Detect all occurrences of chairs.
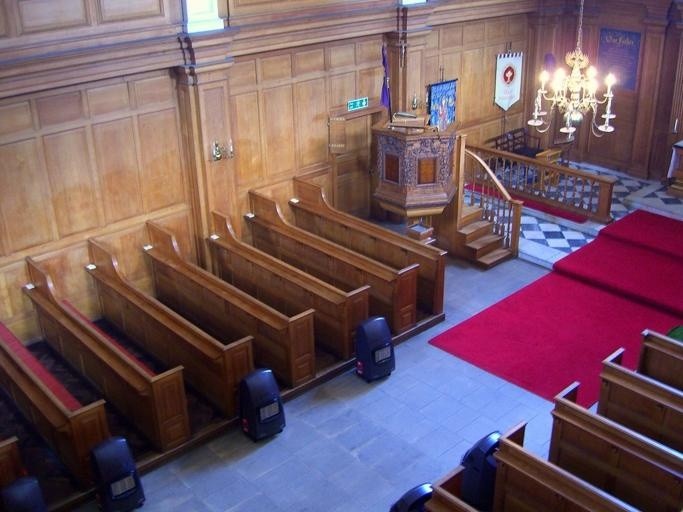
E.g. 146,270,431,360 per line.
513,129,541,175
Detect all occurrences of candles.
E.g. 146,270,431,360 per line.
673,117,677,131
533,64,613,129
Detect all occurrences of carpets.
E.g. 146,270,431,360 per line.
428,209,683,409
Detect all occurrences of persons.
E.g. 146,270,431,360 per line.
435,96,450,131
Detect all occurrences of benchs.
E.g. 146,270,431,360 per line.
388,328,683,512
0,177,447,512
